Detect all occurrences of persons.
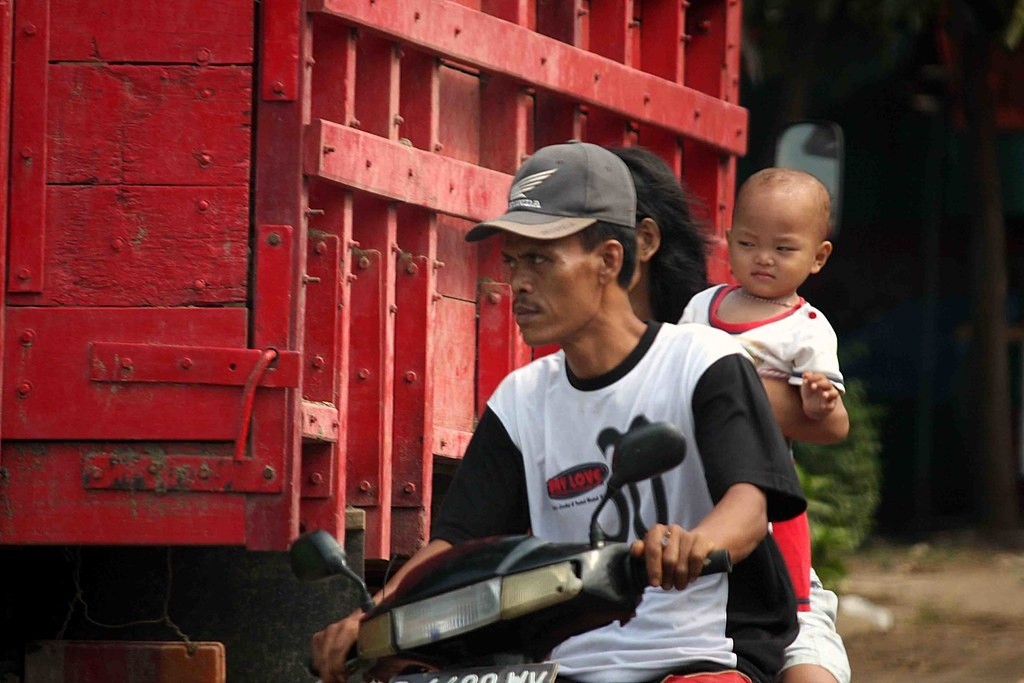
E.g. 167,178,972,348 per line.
309,140,852,683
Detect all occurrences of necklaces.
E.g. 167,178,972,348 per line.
736,286,797,308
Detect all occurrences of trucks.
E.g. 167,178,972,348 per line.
0,0,845,683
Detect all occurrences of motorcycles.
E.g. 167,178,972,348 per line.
285,526,730,683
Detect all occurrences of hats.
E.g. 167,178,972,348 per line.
462,139,638,238
